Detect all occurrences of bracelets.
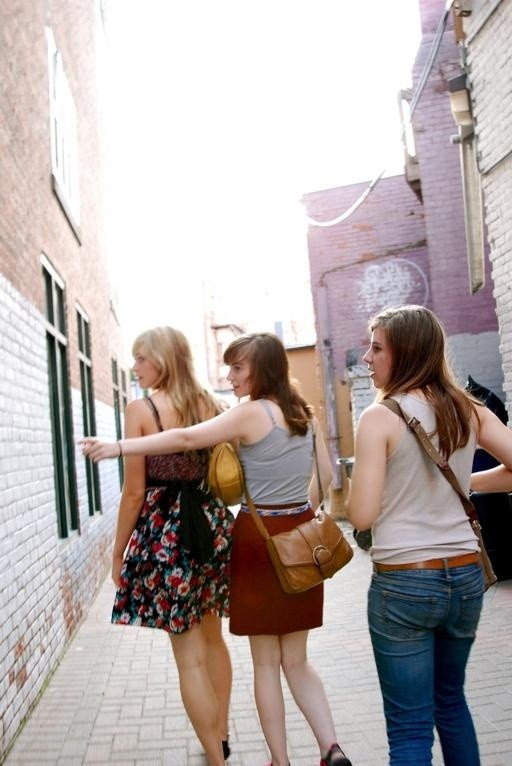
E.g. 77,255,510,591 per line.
117,440,123,458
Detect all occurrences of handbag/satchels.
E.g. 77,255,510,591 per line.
266,510,353,595
470,512,498,593
207,441,244,506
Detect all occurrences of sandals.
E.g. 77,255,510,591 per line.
321,743,352,766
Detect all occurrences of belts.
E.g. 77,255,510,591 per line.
373,552,479,570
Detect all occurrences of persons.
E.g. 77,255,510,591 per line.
110,325,234,766
76,332,353,766
346,301,512,765
